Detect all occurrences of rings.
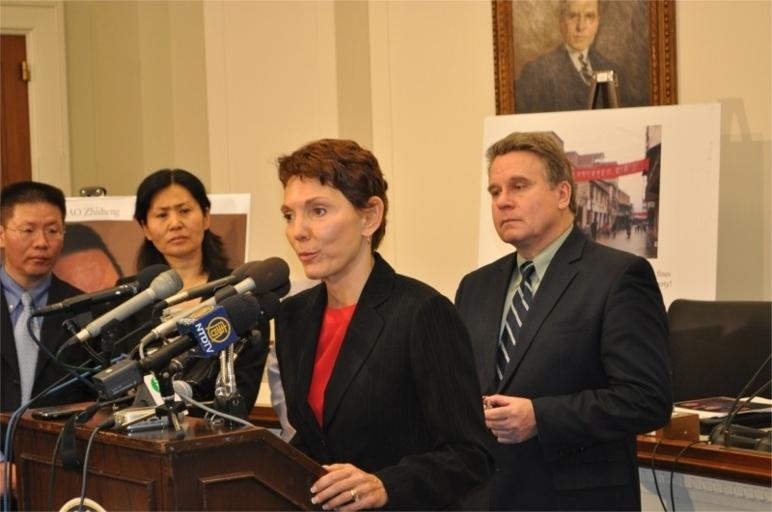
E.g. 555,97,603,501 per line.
351,490,359,502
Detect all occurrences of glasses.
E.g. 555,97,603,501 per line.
6,224,65,240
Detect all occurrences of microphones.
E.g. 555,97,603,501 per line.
30,262,170,318
155,259,261,310
150,256,290,340
75,269,185,344
272,281,291,300
708,352,772,452
167,291,282,374
92,294,264,403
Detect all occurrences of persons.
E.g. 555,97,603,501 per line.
51,222,125,293
515,0,631,115
455,132,674,512
105,168,270,426
0,181,106,512
274,138,493,511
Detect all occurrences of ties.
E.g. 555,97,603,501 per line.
13,292,40,408
494,261,535,389
579,54,593,86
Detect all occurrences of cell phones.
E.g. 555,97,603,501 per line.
31,405,85,421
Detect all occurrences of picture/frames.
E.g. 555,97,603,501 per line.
491,0,678,119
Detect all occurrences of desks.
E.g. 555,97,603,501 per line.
637,402,771,512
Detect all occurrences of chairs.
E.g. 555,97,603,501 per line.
666,297,772,403
